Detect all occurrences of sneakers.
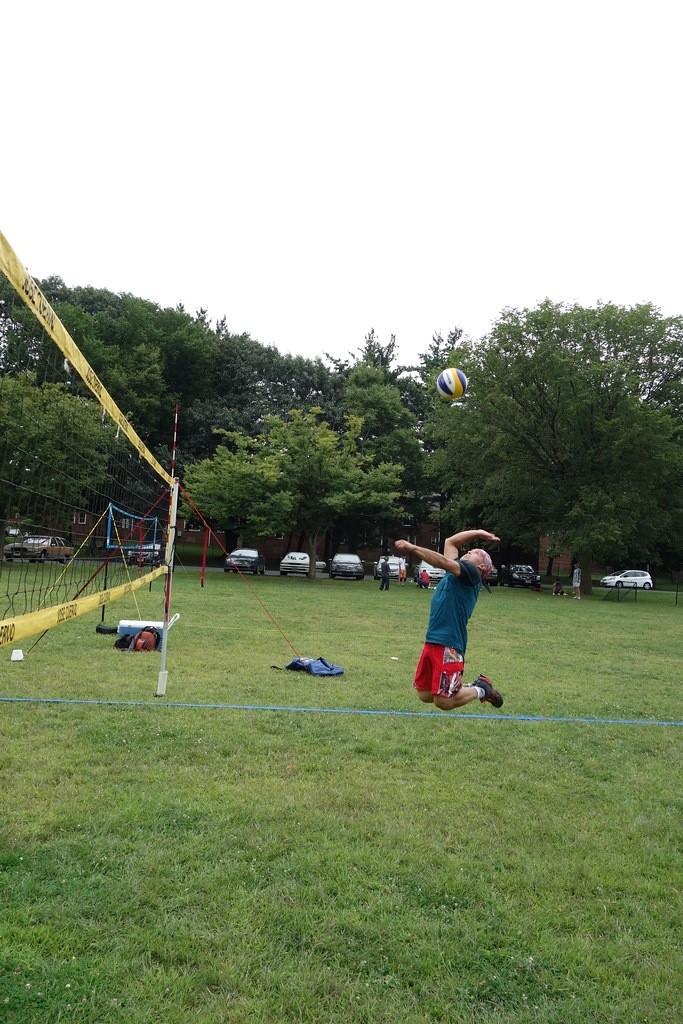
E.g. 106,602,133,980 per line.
472,674,503,708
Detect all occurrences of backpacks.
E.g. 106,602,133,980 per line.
115,632,135,648
133,625,161,652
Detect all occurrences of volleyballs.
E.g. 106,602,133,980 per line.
436,367,468,400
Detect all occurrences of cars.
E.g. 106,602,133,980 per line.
279,552,310,577
8,529,21,537
599,570,653,590
373,555,408,582
500,563,542,589
223,547,266,574
328,552,366,580
2,535,75,564
413,559,446,583
127,542,166,567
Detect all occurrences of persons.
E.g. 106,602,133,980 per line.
394,529,503,710
533,580,543,591
552,578,564,596
482,574,493,593
398,554,407,586
572,564,581,600
416,570,429,588
379,556,391,592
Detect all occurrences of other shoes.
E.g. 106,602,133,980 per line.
573,596,577,598
578,596,580,600
558,593,560,595
552,592,555,596
